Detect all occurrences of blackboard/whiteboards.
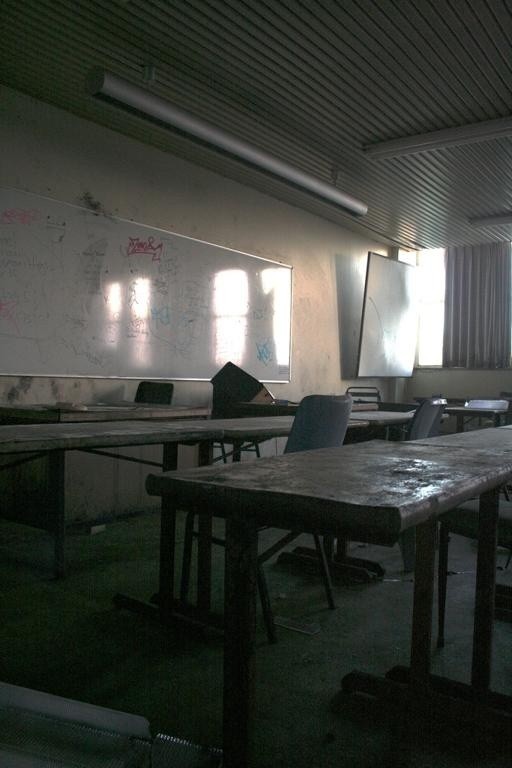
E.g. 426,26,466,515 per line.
1,181,295,385
353,250,427,380
